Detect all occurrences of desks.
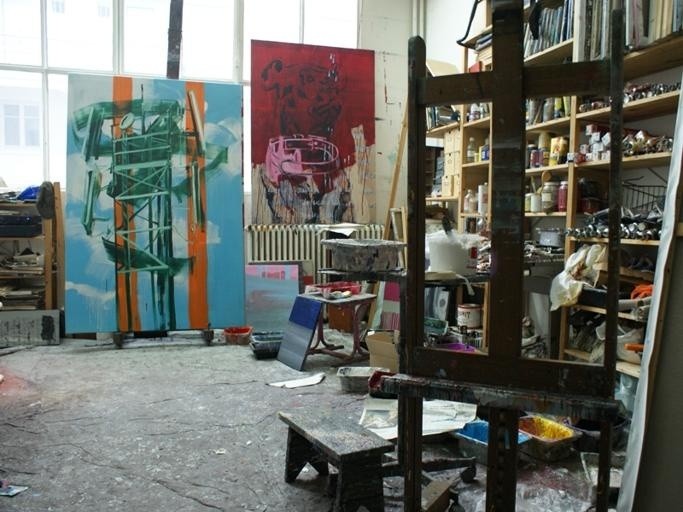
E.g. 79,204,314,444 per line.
296,291,378,365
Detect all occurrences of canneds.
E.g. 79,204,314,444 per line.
541,181,568,211
530,150,539,168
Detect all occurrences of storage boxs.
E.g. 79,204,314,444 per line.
308,281,362,296
318,239,408,271
365,331,400,374
337,367,398,398
222,326,285,360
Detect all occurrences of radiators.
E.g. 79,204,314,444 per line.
246,223,393,321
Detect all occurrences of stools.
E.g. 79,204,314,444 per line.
277,408,395,512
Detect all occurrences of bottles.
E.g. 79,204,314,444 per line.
525,98,570,212
462,102,492,216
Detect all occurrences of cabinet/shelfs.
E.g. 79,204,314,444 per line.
379,2,683,512
0,181,65,346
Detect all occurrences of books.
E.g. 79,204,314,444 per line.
0,248,44,311
0,192,20,215
426,106,454,130
523,0,683,63
475,32,493,66
